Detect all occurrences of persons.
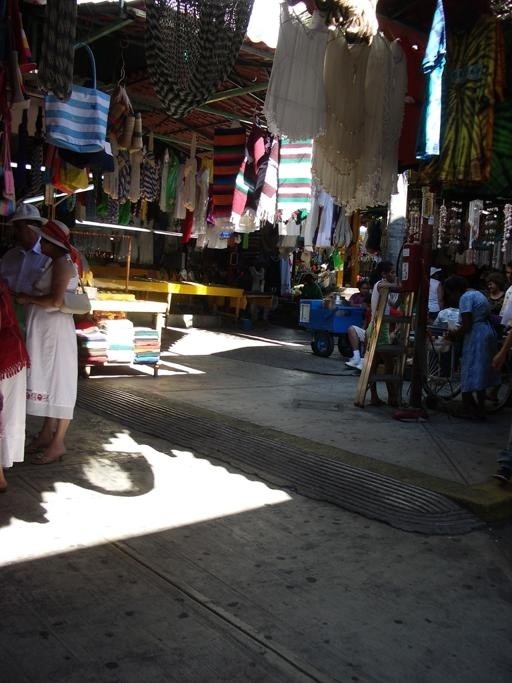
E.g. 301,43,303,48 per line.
344,260,512,482
0,277,30,492
15,218,79,466
300,273,323,298
0,203,52,333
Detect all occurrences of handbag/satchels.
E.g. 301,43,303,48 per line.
60,292,91,314
0,84,143,216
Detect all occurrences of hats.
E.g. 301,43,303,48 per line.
8,204,71,250
430,266,442,276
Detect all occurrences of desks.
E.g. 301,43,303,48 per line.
88,263,243,332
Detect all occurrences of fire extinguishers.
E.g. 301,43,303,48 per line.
396,231,420,291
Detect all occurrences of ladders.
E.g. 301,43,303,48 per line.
354,283,416,409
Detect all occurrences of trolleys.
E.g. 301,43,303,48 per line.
300,299,368,358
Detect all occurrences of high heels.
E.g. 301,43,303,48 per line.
25,443,65,465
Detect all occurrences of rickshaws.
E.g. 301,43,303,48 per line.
422,326,512,414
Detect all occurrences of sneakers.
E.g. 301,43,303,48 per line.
344,356,364,370
492,467,512,481
